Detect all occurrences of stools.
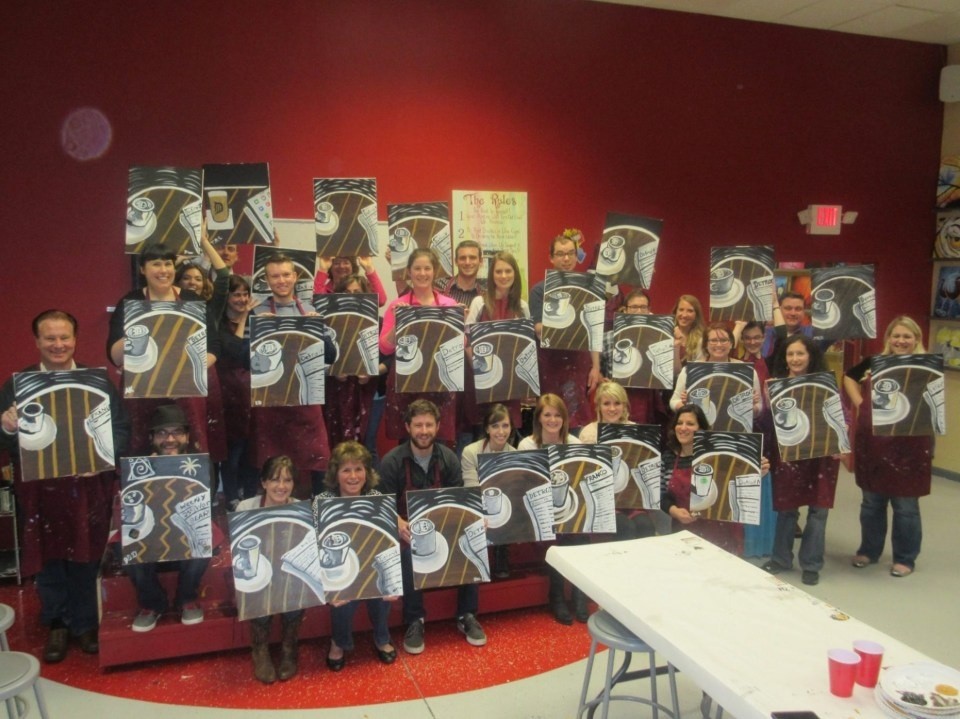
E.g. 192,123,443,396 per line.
0,604,17,651
0,652,49,719
576,608,679,719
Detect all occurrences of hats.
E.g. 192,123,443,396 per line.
146,406,190,427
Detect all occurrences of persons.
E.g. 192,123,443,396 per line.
0,307,129,663
107,217,935,685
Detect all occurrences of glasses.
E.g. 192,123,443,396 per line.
552,250,576,257
706,338,732,344
626,304,650,311
151,428,188,437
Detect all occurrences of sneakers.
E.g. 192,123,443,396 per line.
180,602,203,625
132,607,161,633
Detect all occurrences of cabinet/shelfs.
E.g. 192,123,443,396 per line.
0,484,22,586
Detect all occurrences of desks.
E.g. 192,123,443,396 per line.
545,526,960,719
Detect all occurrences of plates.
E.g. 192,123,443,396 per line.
875,661,960,719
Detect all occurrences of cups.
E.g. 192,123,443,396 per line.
853,640,884,688
827,648,860,698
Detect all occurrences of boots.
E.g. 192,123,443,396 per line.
277,613,301,679
251,621,276,683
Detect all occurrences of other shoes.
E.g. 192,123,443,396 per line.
403,617,425,653
891,563,914,575
801,569,819,585
852,554,872,567
762,559,794,575
457,613,488,645
492,568,509,579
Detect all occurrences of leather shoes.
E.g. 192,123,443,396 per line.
45,628,97,661
372,641,398,664
328,645,345,670
569,596,590,622
548,594,573,625
